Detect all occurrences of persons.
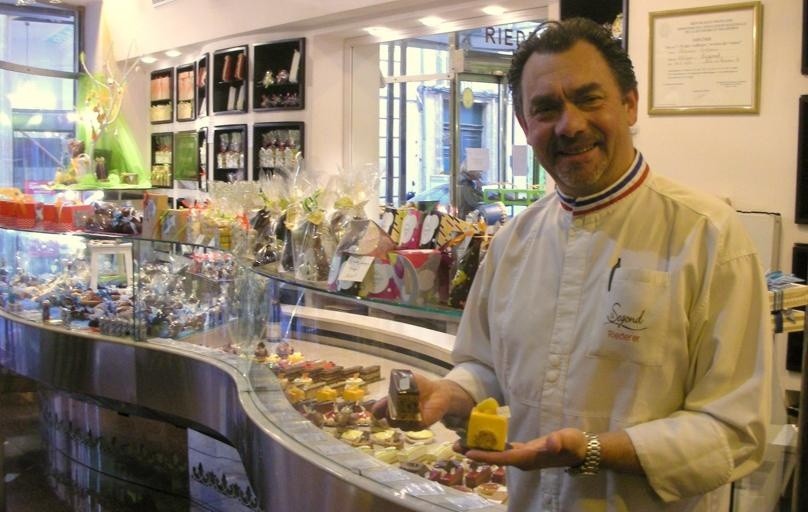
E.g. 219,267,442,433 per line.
456,157,484,222
360,18,776,512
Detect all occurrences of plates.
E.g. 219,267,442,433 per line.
458,435,512,455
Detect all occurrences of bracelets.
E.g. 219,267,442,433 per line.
564,430,603,480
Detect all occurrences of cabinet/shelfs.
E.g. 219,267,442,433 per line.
148,37,305,192
